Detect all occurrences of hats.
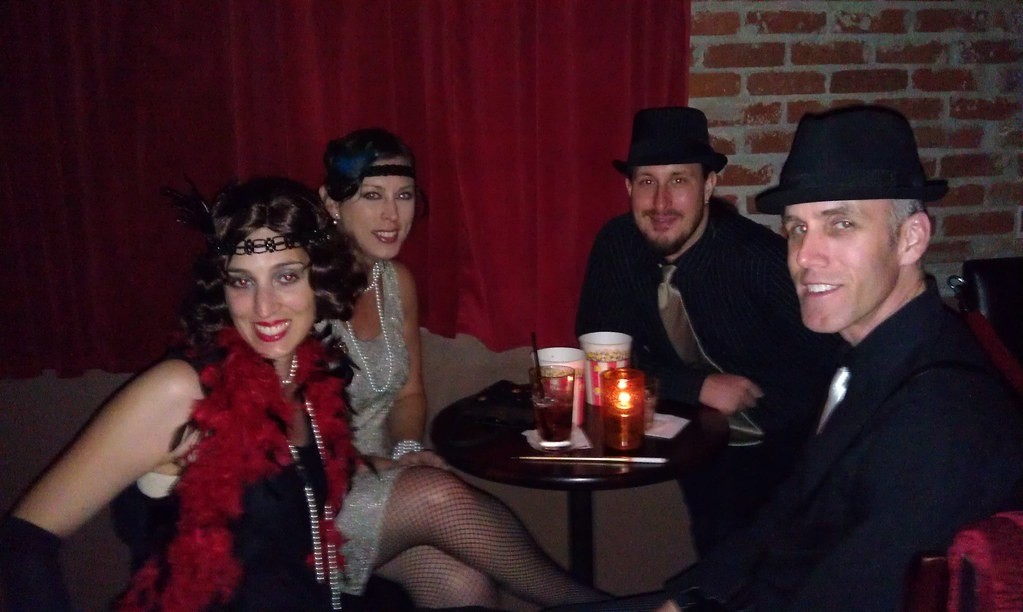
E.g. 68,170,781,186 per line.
613,106,728,173
755,104,948,215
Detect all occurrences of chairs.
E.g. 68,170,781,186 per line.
904,307,1023,612
948,257,1023,373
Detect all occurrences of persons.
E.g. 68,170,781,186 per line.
544,106,1022,612
318,130,616,608
575,108,852,560
0,176,413,612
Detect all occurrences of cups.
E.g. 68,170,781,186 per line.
527,332,660,451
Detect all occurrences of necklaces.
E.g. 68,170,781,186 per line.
279,353,342,610
345,261,392,392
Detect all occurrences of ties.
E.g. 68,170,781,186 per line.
815,367,854,435
658,265,765,448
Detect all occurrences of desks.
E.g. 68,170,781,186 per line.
428,379,680,590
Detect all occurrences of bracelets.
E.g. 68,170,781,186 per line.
393,441,422,460
